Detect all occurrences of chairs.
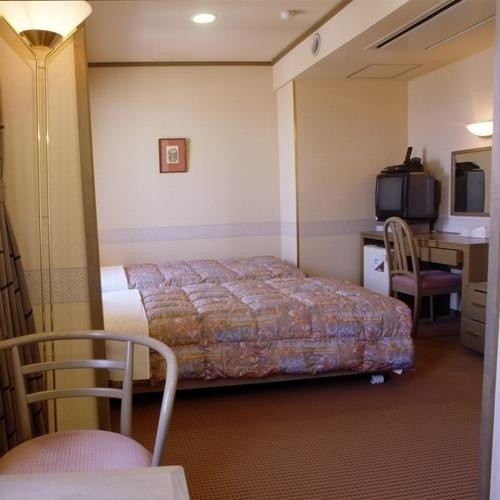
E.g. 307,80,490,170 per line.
380,217,463,340
1,328,179,475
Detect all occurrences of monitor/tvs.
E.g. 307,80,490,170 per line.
455,170,484,212
375,173,434,222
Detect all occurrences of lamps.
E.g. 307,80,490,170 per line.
1,0,94,435
466,121,494,138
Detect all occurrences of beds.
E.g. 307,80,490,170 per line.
99,256,306,293
99,277,411,399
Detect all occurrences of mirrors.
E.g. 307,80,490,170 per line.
450,145,492,217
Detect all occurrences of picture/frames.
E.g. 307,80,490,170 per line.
159,138,187,173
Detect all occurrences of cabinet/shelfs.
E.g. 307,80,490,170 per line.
459,281,487,353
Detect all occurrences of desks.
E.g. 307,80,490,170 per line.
360,231,488,299
1,464,191,500
